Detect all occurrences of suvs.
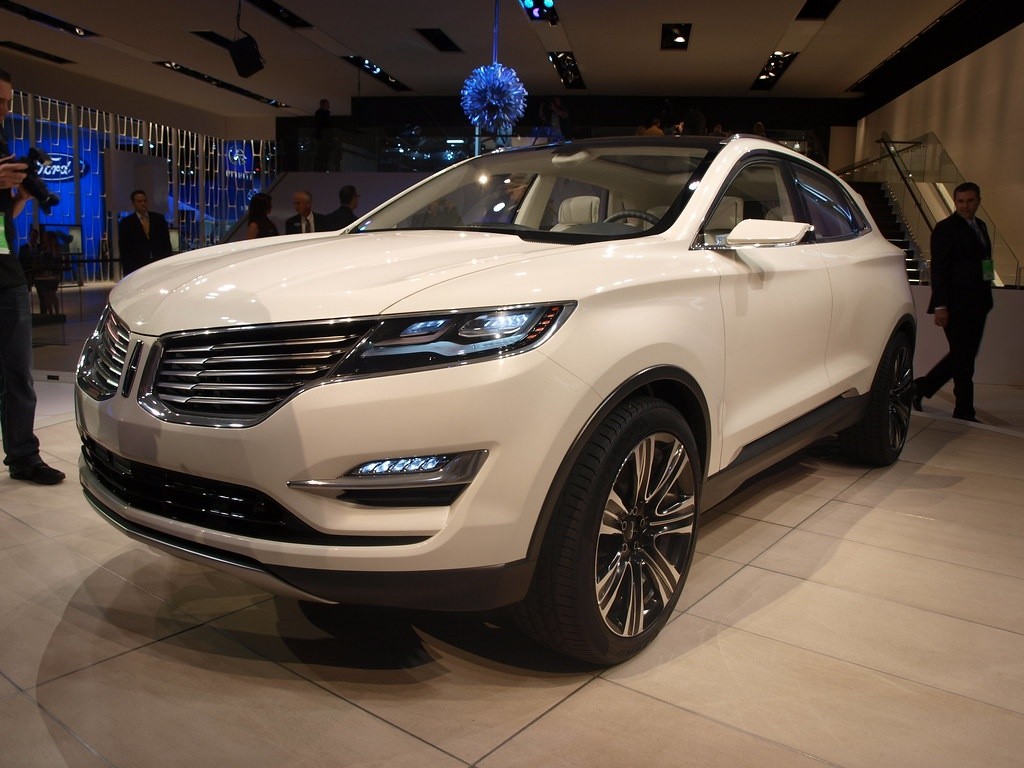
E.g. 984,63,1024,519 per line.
71,134,918,667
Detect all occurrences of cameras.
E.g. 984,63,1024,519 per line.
8,147,61,215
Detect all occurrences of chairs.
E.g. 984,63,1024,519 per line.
549,194,600,233
700,196,745,246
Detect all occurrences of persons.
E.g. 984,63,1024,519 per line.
285,190,328,234
246,193,279,239
0,65,66,484
19,229,60,322
119,190,173,278
327,185,361,234
709,123,725,137
913,183,994,423
643,118,665,135
665,120,684,136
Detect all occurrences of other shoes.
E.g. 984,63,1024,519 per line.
964,418,980,423
912,382,923,412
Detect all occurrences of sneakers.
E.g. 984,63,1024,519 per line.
10,462,65,485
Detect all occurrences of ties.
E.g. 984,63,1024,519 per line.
305,218,310,233
141,215,149,238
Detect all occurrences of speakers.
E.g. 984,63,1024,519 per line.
229,36,264,78
